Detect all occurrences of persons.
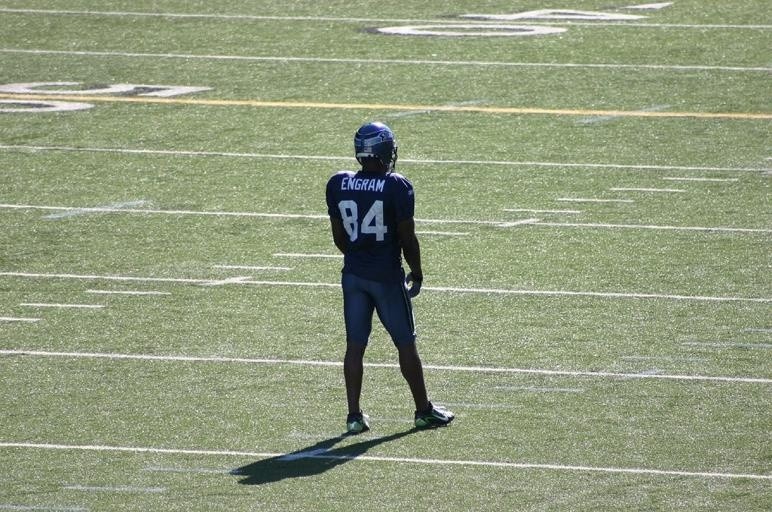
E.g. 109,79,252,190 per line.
325,123,454,433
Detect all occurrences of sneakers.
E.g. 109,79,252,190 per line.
414,401,456,430
347,409,370,433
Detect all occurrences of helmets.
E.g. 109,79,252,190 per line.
354,121,399,172
